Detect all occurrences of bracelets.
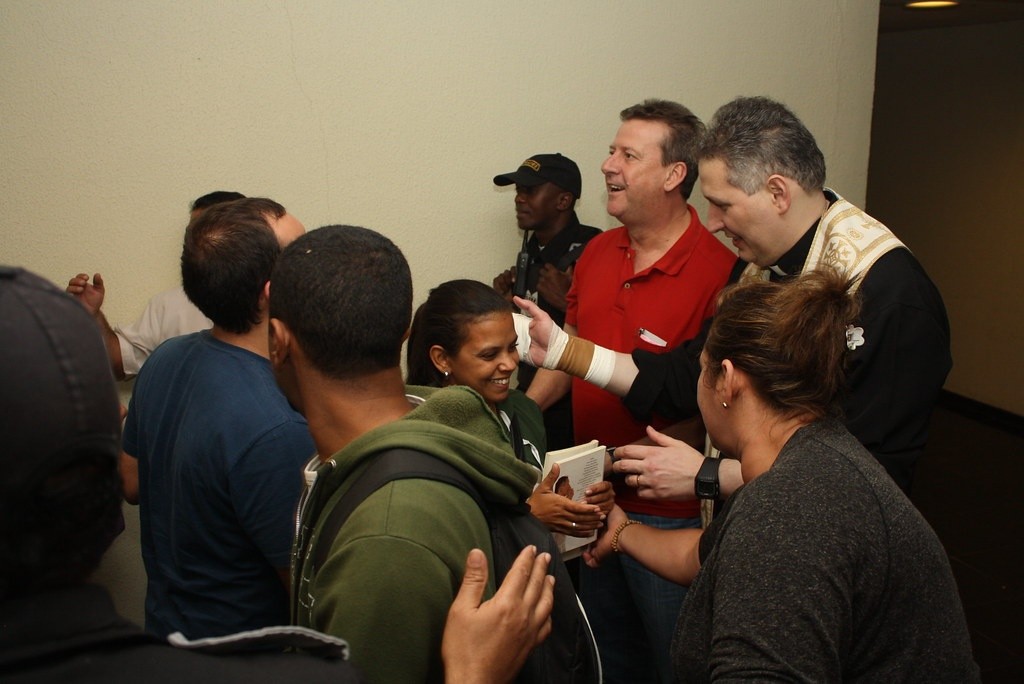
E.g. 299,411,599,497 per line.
610,519,642,551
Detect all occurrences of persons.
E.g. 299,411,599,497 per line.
267,224,540,684
493,153,603,451
65,191,248,383
406,278,616,539
102,197,316,636
584,263,981,684
509,97,953,501
552,476,574,553
527,99,738,684
0,264,557,684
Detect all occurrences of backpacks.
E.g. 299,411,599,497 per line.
305,447,602,684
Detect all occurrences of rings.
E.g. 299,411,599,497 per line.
637,475,640,485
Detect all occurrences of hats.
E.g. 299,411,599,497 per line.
0,267,123,575
493,152,582,199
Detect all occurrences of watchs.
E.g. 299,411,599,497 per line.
694,457,722,500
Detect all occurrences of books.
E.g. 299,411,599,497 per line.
542,439,606,562
